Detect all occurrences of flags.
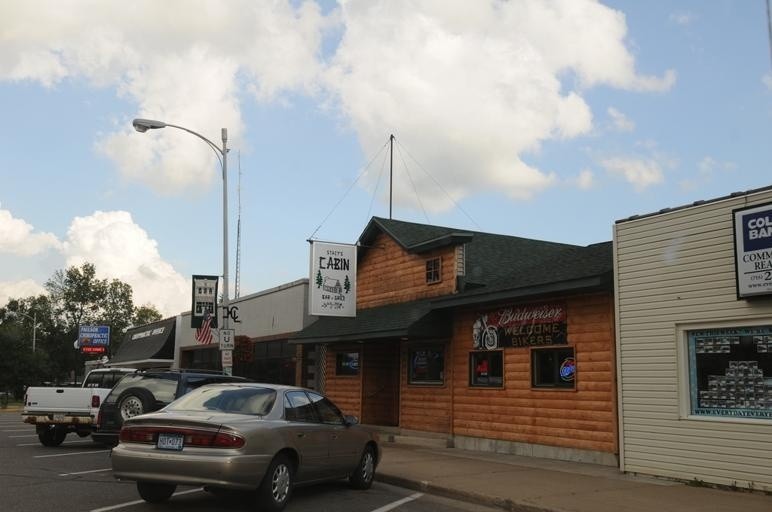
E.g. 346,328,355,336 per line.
195,310,214,346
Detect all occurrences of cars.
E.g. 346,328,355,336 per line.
111,383,383,511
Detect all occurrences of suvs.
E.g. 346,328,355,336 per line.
91,367,257,444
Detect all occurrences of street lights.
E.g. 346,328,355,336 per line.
6,312,37,352
132,119,233,376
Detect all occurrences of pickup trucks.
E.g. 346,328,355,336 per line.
19,367,148,448
